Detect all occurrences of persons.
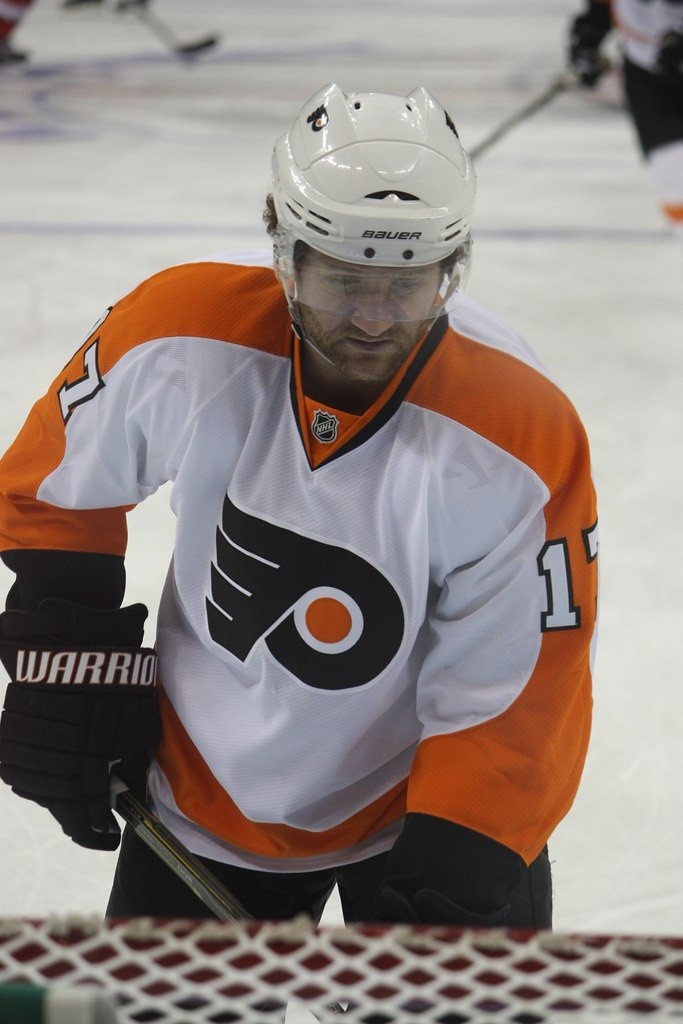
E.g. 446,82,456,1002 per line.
0,82,599,931
566,0,683,223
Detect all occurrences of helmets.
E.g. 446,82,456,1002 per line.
271,85,479,275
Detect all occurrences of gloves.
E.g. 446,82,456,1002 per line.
0,602,163,853
571,45,608,88
656,31,682,72
